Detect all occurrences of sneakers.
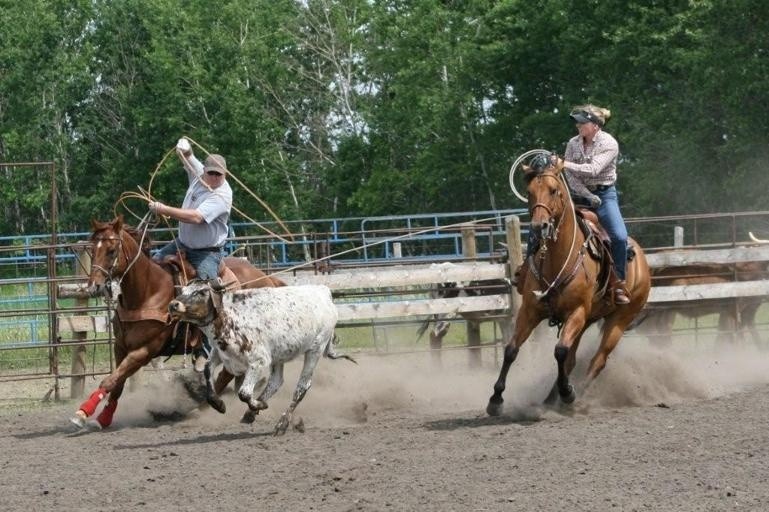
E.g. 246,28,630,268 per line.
605,287,630,305
192,336,213,374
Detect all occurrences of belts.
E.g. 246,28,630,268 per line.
192,246,220,253
586,185,609,192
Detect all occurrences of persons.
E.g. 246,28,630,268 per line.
149,137,233,373
512,105,630,304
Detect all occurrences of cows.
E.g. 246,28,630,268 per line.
166,275,359,434
652,230,769,354
415,240,531,370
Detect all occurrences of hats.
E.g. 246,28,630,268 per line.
568,110,604,128
205,153,227,174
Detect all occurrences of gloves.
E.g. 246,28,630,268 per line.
590,196,603,209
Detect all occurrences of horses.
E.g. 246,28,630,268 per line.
486,151,654,418
66,213,288,435
